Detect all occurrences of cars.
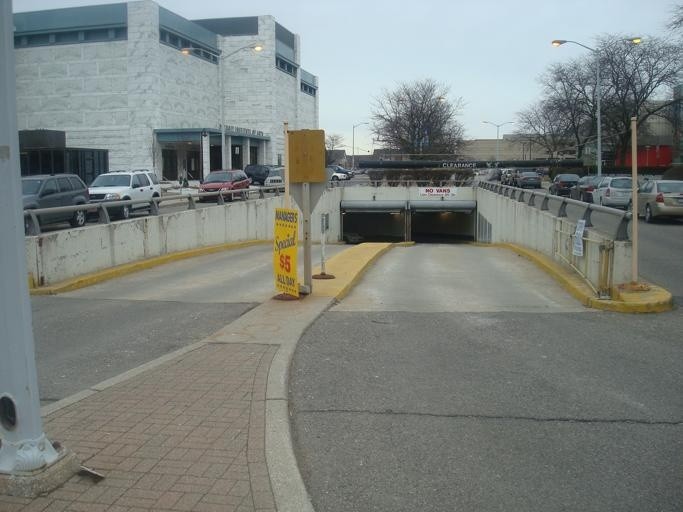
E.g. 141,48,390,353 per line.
244,164,354,193
478,169,640,210
628,178,683,224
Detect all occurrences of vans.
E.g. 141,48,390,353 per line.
197,168,252,203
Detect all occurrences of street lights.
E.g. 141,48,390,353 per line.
395,97,447,154
550,36,644,176
352,122,370,170
481,121,516,167
179,44,264,169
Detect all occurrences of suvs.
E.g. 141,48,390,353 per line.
85,167,163,219
21,171,92,237
